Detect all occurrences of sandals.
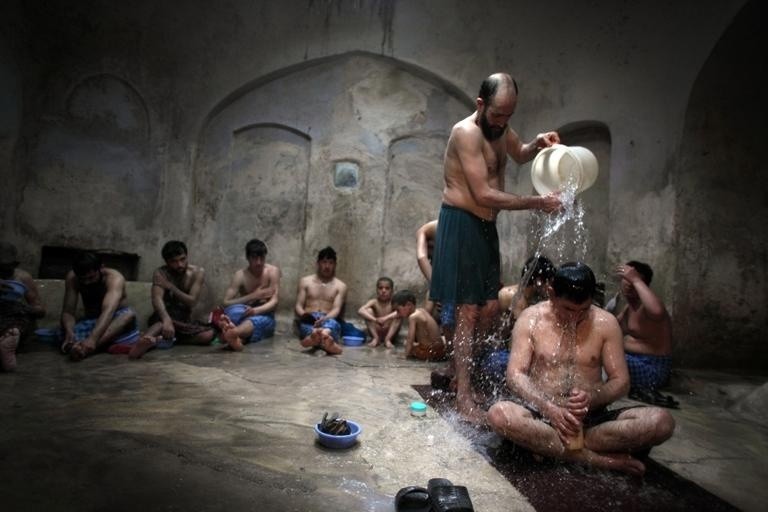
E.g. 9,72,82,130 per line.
629,382,680,408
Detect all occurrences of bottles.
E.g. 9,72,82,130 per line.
566,417,584,450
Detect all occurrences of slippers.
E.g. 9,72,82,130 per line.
428,477,476,511
395,485,433,512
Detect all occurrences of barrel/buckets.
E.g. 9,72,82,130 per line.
530,142,599,200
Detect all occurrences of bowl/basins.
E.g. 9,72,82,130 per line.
315,417,361,450
342,335,365,347
33,329,53,342
224,305,246,322
2,280,28,299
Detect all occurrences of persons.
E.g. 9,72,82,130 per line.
218,238,280,351
128,239,218,362
295,246,347,354
60,248,137,362
485,261,677,476
357,277,402,348
1,241,47,372
391,223,681,408
430,71,563,426
